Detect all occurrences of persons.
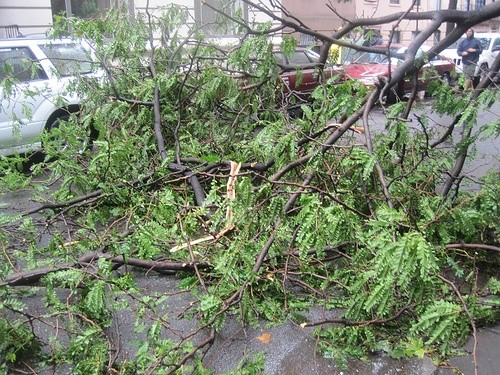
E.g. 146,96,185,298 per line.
457,28,483,97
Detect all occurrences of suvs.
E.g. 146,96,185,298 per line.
0,39,108,169
439,31,500,89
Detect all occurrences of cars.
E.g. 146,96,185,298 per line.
181,47,344,109
339,44,455,108
